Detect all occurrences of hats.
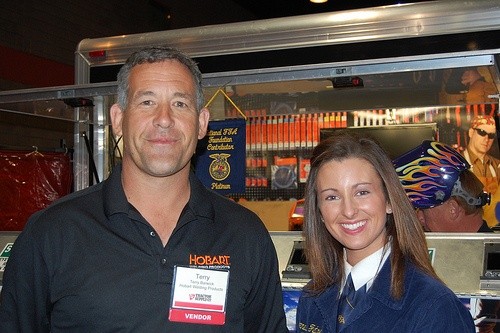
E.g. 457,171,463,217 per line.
470,114,496,129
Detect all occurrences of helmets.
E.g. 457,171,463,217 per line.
392,140,471,208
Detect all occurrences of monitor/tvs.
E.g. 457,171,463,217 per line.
320,122,438,163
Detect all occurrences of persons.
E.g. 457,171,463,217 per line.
0,46,290,333
295,132,476,333
460,114,500,229
390,140,495,233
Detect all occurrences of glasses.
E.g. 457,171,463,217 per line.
472,127,496,139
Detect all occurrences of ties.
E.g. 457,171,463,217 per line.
336,273,366,333
